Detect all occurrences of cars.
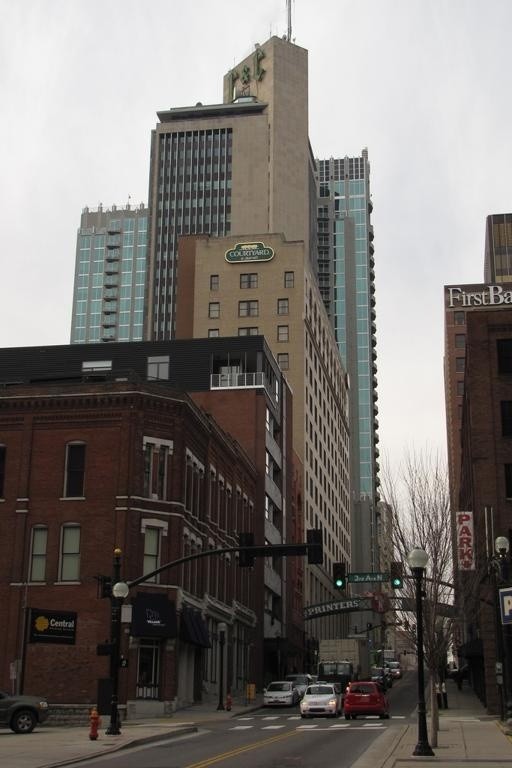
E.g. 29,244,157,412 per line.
262,634,403,720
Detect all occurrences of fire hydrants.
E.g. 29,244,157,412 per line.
88,706,103,740
226,693,232,710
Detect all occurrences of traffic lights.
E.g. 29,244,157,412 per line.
333,561,346,589
390,560,403,587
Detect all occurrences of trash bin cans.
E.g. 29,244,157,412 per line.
247,684,254,698
436,692,448,709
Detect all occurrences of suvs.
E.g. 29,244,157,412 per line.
0,688,50,734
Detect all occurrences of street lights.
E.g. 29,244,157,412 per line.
406,546,439,755
216,621,228,709
491,533,510,721
104,580,131,735
